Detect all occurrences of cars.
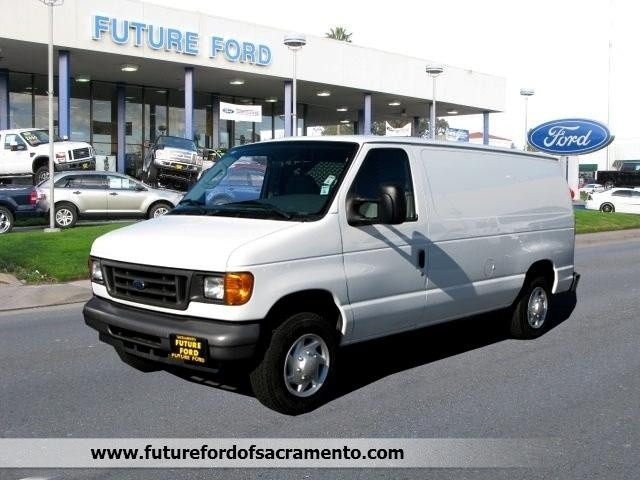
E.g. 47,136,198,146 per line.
0,128,268,235
576,171,640,216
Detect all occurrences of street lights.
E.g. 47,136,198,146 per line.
426,62,444,139
519,86,536,150
41,0,66,233
284,30,309,137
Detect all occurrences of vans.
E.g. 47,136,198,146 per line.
86,133,580,415
